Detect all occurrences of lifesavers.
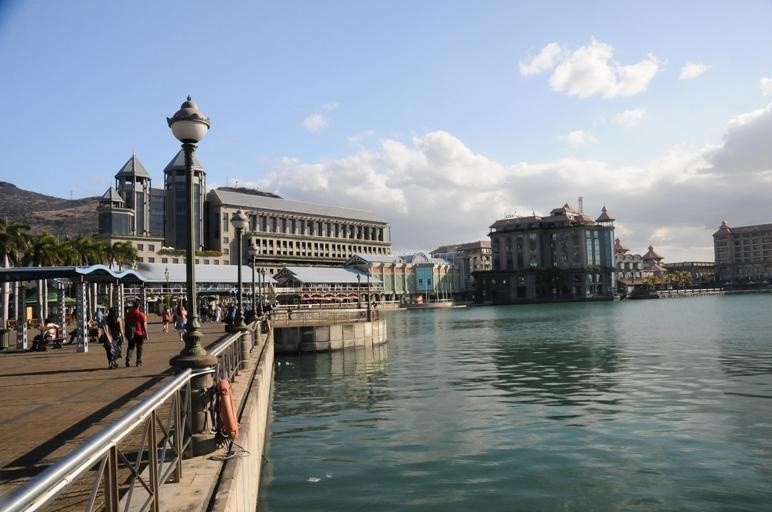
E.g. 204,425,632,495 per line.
217,380,238,440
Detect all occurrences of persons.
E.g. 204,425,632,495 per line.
162,298,236,342
124,301,148,367
102,307,123,368
94,307,109,323
30,319,60,351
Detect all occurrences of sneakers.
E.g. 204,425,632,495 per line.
180,338,182,342
135,361,143,366
110,362,118,368
125,362,131,367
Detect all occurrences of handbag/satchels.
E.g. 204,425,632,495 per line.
124,323,135,341
109,346,122,360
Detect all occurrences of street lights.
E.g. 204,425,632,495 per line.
230,207,266,323
167,95,211,356
357,272,362,307
